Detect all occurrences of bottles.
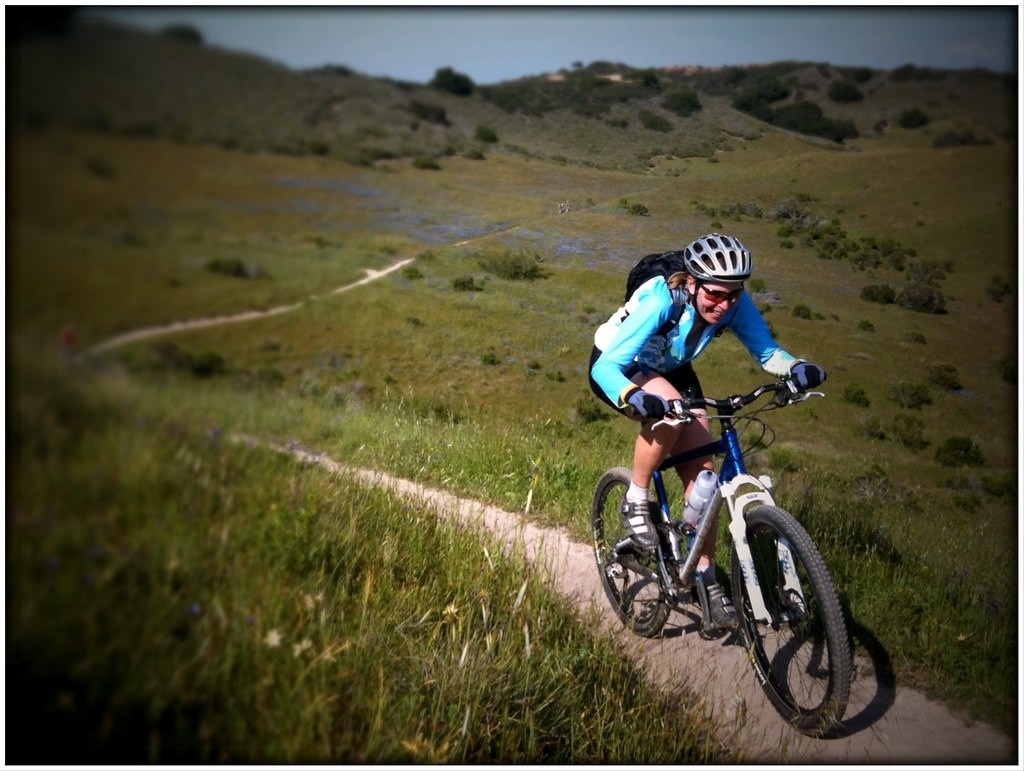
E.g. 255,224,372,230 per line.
682,470,717,527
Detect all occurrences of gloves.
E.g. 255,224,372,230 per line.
791,362,827,394
625,387,670,418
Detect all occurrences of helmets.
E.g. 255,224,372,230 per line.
684,232,752,282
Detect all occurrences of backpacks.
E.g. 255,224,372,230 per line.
626,249,689,337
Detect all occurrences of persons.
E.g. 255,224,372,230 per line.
588,232,828,629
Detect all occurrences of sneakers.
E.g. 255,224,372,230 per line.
707,584,735,625
618,497,659,550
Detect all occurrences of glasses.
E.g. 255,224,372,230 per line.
701,283,744,302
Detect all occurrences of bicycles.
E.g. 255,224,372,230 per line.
591,377,852,739
